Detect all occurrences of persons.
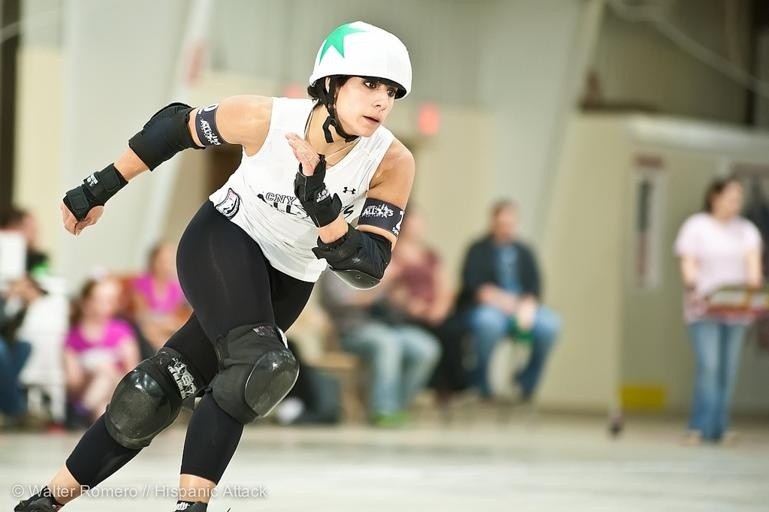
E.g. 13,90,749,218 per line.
0,198,563,428
14,20,418,512
672,176,765,450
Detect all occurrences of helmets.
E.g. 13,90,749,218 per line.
307,18,413,98
